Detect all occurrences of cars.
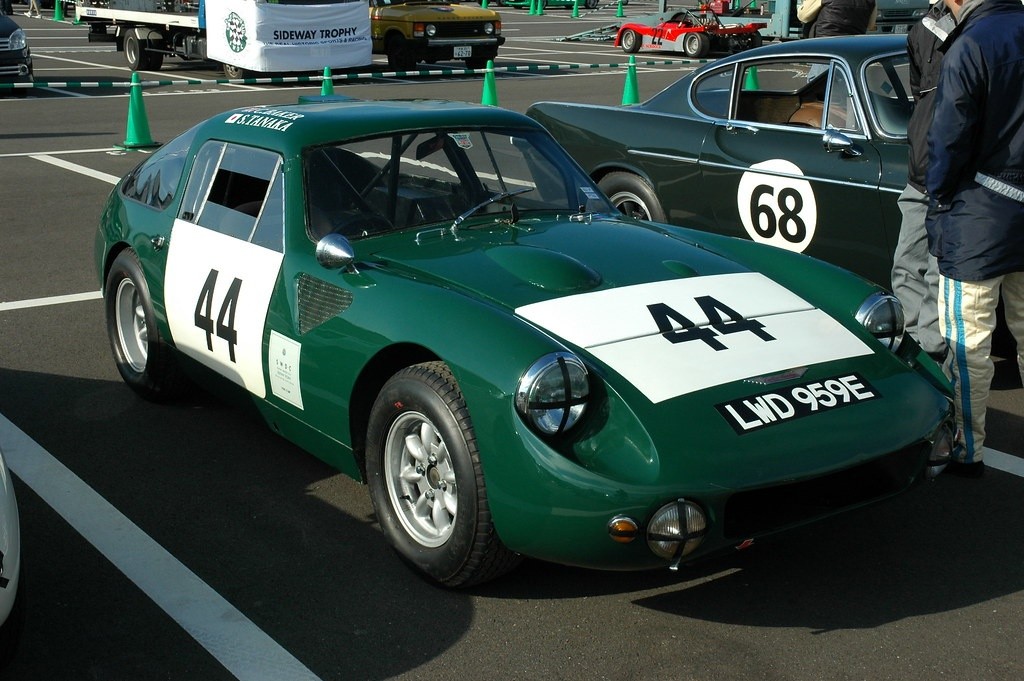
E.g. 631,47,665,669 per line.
0,8,38,97
97,97,959,591
526,33,1023,361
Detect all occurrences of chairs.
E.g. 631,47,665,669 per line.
788,100,849,129
222,199,334,236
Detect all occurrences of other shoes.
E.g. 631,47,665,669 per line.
944,460,985,479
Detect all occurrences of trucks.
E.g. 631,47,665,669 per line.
369,0,509,73
74,1,374,84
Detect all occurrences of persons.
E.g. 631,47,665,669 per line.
891,0,1023,498
796,0,877,104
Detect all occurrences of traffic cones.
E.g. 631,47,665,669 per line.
621,54,641,106
480,60,499,108
481,0,488,10
113,71,164,152
744,64,761,90
733,0,758,14
613,0,626,18
51,0,89,26
321,67,334,97
527,0,547,16
